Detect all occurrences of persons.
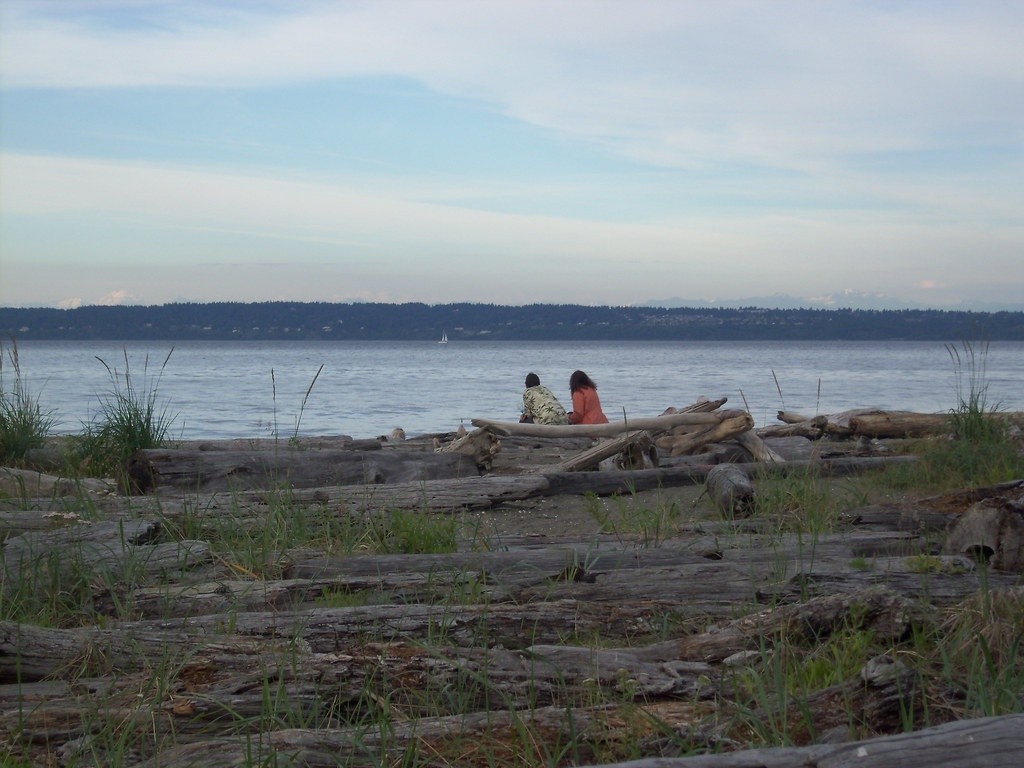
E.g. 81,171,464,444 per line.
567,370,609,424
519,373,568,425
